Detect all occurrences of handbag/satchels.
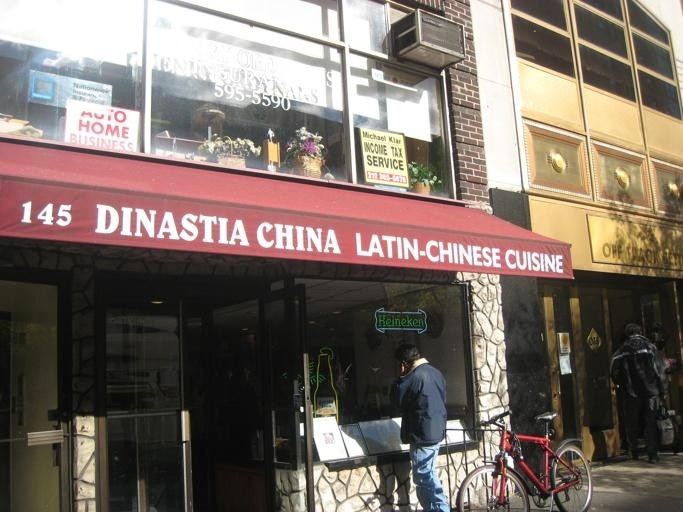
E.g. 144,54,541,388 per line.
654,399,679,452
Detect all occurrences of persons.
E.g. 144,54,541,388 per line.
611,322,670,463
390,344,451,512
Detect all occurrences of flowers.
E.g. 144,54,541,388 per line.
407,161,443,193
285,126,328,164
197,132,262,158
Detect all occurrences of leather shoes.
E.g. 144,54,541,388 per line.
649,456,658,464
625,455,640,461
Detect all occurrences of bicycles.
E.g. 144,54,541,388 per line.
457,409,591,511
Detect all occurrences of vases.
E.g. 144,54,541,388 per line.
218,157,246,170
414,182,431,196
290,156,322,180
